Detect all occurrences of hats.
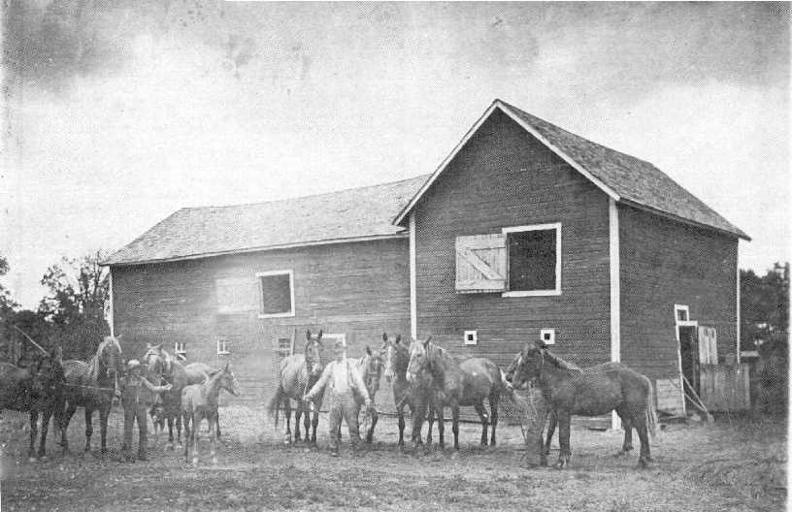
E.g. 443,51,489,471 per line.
126,359,142,372
332,340,347,354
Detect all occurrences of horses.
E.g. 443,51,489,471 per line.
266,327,328,453
51,330,126,461
177,363,241,468
514,343,657,473
0,343,74,463
381,330,437,448
504,343,635,456
404,336,504,453
143,340,223,453
346,345,388,445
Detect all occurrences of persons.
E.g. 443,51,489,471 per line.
111,359,173,460
303,340,372,457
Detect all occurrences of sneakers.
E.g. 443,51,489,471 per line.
119,455,128,462
332,449,337,456
139,455,151,460
